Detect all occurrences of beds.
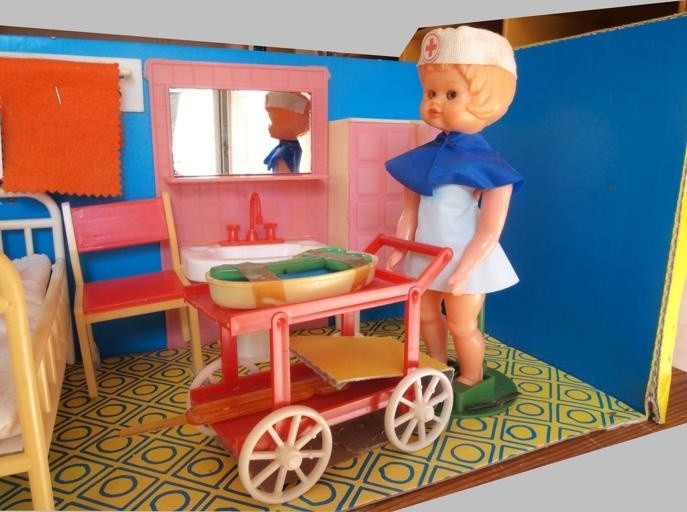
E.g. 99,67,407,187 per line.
1,185,76,511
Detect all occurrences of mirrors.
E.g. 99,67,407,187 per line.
167,88,312,178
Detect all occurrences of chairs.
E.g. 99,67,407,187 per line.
61,190,205,399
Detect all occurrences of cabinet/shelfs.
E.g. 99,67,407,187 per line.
328,118,443,252
